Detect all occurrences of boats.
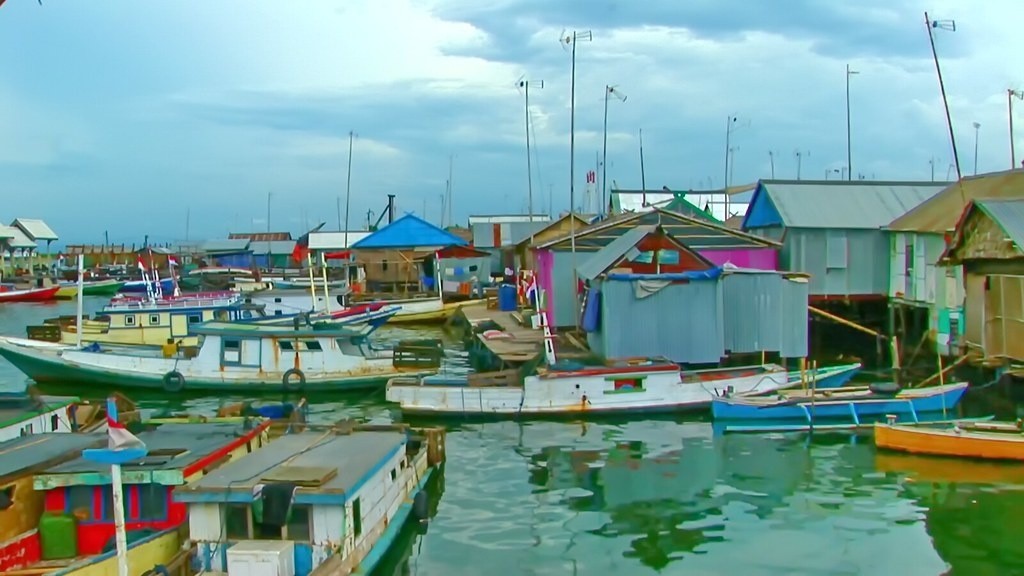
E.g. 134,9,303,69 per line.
0,248,500,352
136,422,445,576
0,390,145,482
384,359,863,424
0,316,441,397
710,359,968,434
0,398,311,576
874,413,1023,462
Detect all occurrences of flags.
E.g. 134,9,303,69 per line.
168,254,178,266
138,256,151,273
